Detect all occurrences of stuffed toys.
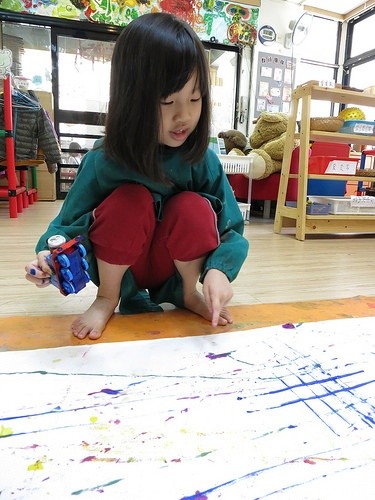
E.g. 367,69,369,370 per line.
229,111,299,180
218,130,252,158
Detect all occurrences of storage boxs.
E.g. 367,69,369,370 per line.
286,195,374,218
309,156,360,174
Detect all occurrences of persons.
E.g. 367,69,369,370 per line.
23,13,250,340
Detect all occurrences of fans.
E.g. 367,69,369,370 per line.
284,12,314,49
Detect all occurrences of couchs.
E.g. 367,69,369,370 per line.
231,144,299,218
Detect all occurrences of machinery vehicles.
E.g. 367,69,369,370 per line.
43,234,92,297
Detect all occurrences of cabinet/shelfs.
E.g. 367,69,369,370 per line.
274,79,375,242
213,154,251,222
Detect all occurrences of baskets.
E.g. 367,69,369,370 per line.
310,118,345,132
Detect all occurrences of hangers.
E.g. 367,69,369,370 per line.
0,88,44,113
